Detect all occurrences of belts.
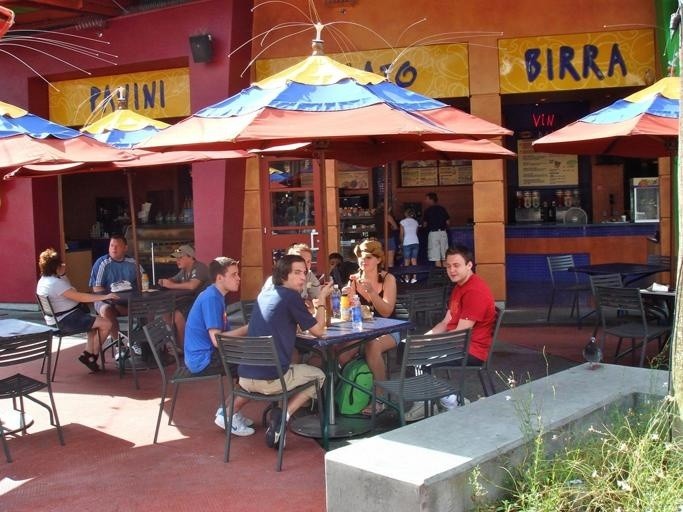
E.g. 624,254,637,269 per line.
430,229,446,232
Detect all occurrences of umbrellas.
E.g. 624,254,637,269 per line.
531,77,681,292
0,88,258,291
131,22,514,328
251,140,513,269
0,100,139,171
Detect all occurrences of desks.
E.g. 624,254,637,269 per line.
387,265,435,286
0,305,59,338
506,222,665,309
292,302,413,348
568,262,671,275
107,286,195,308
394,224,473,287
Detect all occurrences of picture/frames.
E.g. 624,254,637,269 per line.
439,167,474,185
516,136,581,190
401,165,438,188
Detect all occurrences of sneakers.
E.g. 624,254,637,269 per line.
215,404,255,427
127,340,142,355
265,407,290,448
410,278,417,283
215,410,256,437
404,278,410,283
115,346,126,360
404,397,439,422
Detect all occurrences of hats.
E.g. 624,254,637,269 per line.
170,244,195,259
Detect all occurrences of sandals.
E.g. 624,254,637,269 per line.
77,349,100,372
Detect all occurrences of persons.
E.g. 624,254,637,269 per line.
405,247,497,422
37,250,119,372
184,257,255,436
239,255,325,451
337,239,400,416
158,244,210,359
399,206,421,283
423,192,451,267
381,203,398,269
262,243,334,366
89,234,144,356
329,252,343,272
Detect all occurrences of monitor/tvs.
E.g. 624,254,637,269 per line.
64,212,95,240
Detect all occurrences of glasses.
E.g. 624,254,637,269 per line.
174,249,183,253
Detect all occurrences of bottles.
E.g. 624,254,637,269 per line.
331,283,341,319
351,295,362,331
141,271,149,291
340,293,351,321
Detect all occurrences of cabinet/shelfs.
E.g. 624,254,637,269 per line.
630,185,662,222
338,163,374,273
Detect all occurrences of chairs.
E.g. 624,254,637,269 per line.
592,282,674,368
546,254,593,330
241,297,260,328
0,329,66,463
588,272,663,367
383,296,409,381
406,286,448,329
35,293,106,383
646,252,677,289
142,316,231,444
424,304,504,419
215,334,326,474
371,326,471,432
117,295,180,391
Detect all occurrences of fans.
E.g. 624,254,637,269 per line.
563,207,588,225
